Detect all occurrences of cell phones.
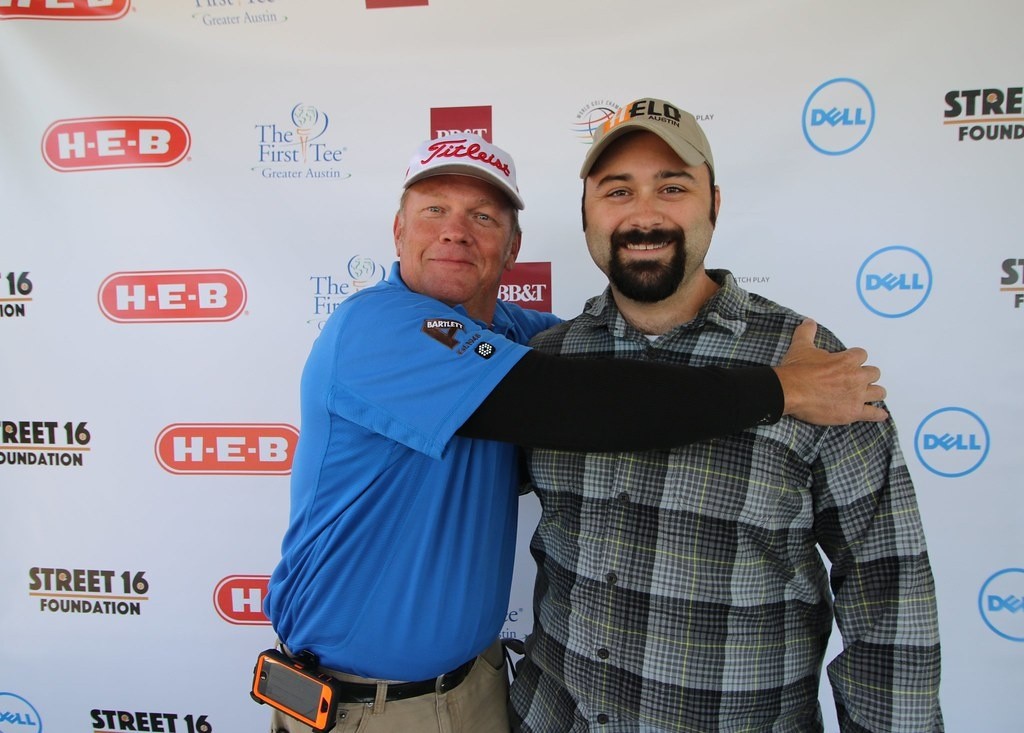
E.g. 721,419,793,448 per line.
253,654,333,731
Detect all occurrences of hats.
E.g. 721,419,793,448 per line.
578,97,716,180
403,132,525,213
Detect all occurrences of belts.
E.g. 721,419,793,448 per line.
275,643,478,703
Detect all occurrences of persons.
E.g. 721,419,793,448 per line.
272,132,890,733
506,97,944,733
